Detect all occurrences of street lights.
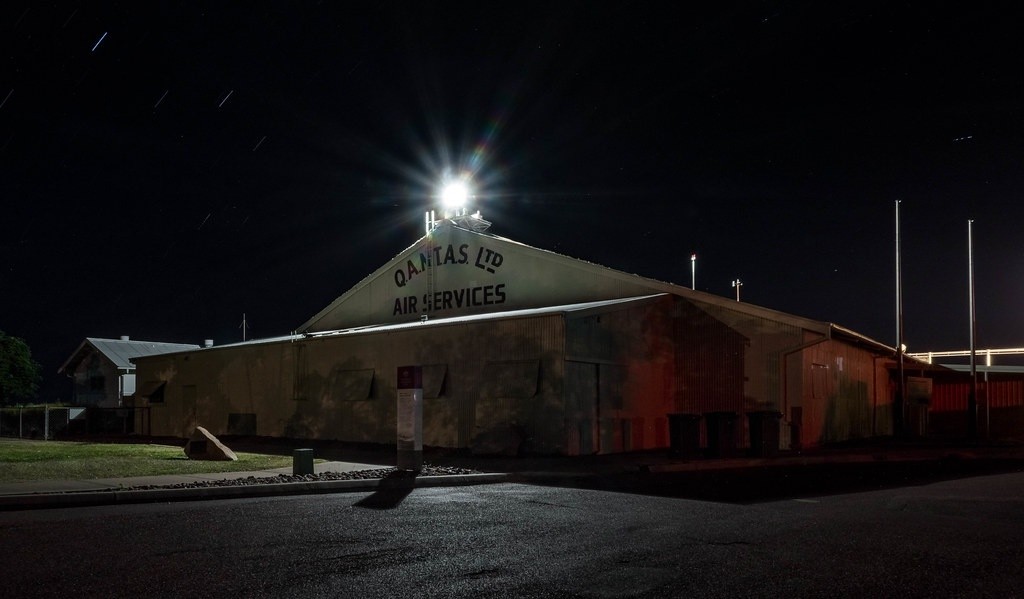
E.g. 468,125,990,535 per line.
691,253,697,290
732,279,744,303
895,199,903,349
968,219,977,383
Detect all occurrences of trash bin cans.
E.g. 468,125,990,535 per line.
666,413,701,458
293,449,314,474
704,410,737,458
745,408,783,458
